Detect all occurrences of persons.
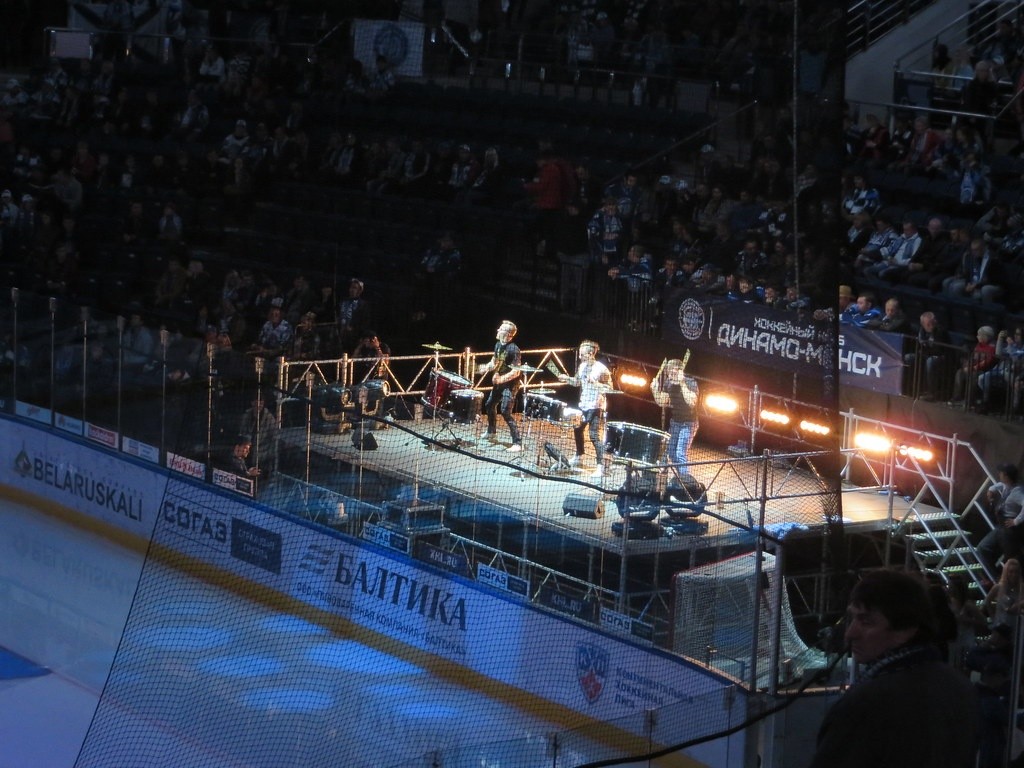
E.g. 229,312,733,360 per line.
479,321,523,452
978,465,1024,571
650,359,699,472
254,306,294,355
951,326,998,403
948,573,984,662
837,295,882,327
904,312,956,394
866,299,908,331
971,623,1012,768
558,340,611,478
0,0,1024,482
350,331,390,378
843,173,879,215
338,279,367,341
814,570,980,768
985,560,1024,634
885,219,951,286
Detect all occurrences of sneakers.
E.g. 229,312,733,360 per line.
571,453,586,465
591,464,605,477
481,432,496,439
506,445,524,453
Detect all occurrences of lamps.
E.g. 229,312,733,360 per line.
353,380,391,430
309,384,352,434
611,477,663,539
658,473,708,536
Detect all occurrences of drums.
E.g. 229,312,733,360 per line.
450,389,484,425
420,367,473,413
524,393,567,424
554,407,582,428
602,421,671,473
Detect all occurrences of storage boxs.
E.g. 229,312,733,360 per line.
378,521,450,571
383,496,444,532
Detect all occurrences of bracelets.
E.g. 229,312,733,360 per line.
376,347,381,351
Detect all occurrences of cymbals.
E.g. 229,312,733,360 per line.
587,385,624,394
528,388,556,393
422,341,453,350
508,362,543,372
